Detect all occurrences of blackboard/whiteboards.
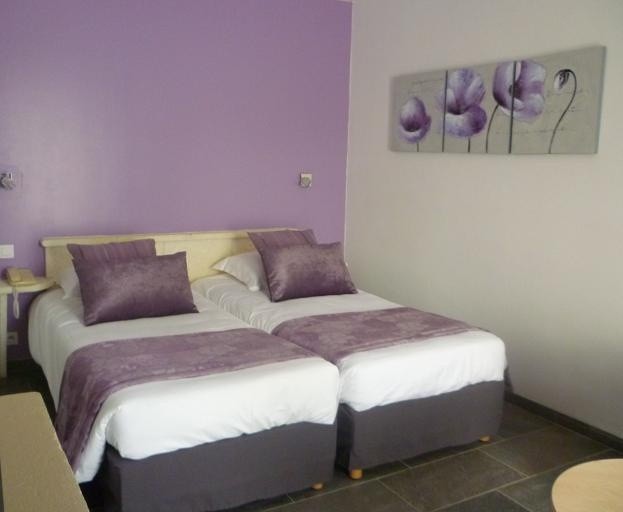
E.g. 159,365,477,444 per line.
3,266,38,287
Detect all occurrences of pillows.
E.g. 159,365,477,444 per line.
208,250,268,290
72,251,199,326
52,263,79,300
67,238,156,263
246,230,318,271
257,241,358,304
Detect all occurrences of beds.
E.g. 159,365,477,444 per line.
28,287,332,512
191,275,510,483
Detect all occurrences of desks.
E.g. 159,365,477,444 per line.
0,272,56,386
